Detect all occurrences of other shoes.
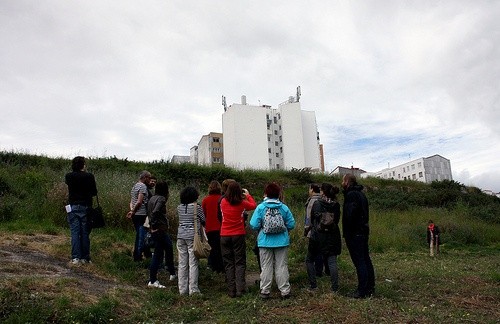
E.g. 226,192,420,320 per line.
346,292,373,298
307,287,320,294
259,293,269,300
281,294,291,300
190,293,204,299
331,289,340,295
229,293,242,297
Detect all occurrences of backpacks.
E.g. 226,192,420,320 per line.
263,208,288,234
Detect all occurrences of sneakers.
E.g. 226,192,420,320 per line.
170,274,178,282
70,258,92,265
148,281,166,290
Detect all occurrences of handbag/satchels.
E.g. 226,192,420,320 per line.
88,193,107,229
311,202,334,233
193,233,212,257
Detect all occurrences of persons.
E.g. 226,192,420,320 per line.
65,156,98,264
305,183,330,278
126,170,157,261
147,181,177,289
201,180,225,274
340,173,375,299
175,186,205,296
426,220,442,256
305,182,342,293
249,183,295,300
217,179,256,296
